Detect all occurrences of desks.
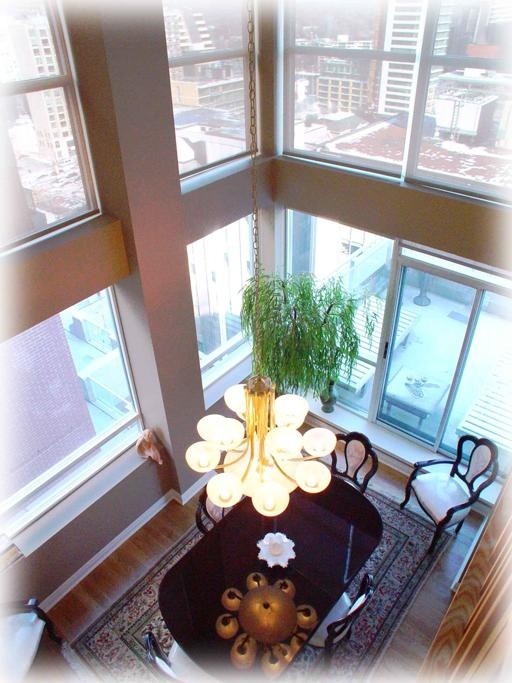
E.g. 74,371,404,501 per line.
158,474,386,683
383,365,451,427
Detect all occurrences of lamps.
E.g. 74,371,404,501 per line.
184,0,338,519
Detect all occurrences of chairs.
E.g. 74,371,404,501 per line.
457,350,511,453
145,630,214,683
1,592,71,683
308,572,374,677
329,429,379,494
309,293,421,396
400,434,499,552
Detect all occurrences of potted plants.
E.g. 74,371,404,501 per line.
241,268,379,414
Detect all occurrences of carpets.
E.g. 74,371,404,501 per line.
69,452,452,683
